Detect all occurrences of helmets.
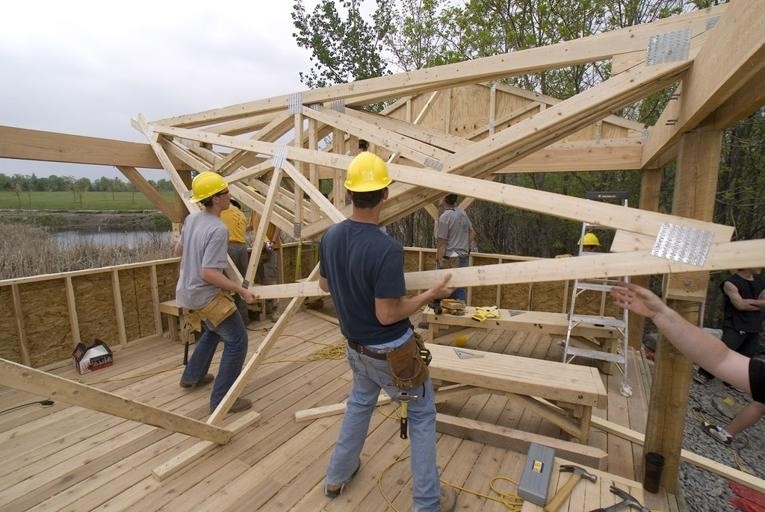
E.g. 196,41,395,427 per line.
229,185,262,210
343,151,393,193
187,170,228,204
577,232,603,247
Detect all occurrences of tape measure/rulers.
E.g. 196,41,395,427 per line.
453,348,484,359
508,311,526,315
420,349,433,367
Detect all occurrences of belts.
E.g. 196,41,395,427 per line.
345,338,389,361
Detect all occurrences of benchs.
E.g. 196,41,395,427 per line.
420,304,621,377
423,340,609,470
158,284,266,346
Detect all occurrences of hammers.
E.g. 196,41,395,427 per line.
542,464,597,512
391,390,419,439
598,485,650,511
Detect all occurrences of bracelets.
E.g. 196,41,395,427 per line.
271,241,276,244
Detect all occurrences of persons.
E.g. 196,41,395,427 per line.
577,233,602,253
692,267,765,393
318,151,458,512
173,172,256,414
434,192,477,303
246,185,282,323
219,197,250,327
342,139,389,235
608,281,765,404
700,400,765,447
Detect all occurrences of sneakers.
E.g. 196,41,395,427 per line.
438,484,457,512
271,308,279,322
179,373,215,387
210,397,252,413
700,421,733,447
692,373,746,394
323,455,361,498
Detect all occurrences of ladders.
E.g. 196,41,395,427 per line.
562,192,632,398
